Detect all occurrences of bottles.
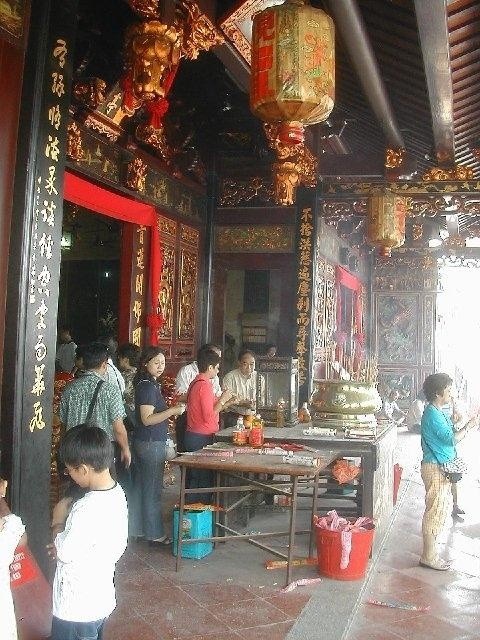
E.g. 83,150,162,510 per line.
232,397,311,449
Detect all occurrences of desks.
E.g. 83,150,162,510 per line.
168,441,341,586
216,421,399,557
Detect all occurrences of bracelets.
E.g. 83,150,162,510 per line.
50,519,64,531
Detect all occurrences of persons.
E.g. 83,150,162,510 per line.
176,343,266,507
49,422,130,640
382,388,478,523
418,372,479,570
56,325,184,547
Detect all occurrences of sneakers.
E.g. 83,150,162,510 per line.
452,509,465,514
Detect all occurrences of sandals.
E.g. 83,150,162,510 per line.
142,536,173,546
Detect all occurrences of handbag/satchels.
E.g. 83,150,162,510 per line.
56,446,70,480
123,402,139,432
175,411,187,433
439,456,472,483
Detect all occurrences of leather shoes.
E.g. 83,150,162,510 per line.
419,554,453,570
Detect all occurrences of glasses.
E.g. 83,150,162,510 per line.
63,463,86,476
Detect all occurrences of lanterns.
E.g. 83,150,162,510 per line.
365,194,408,257
249,0,337,144
